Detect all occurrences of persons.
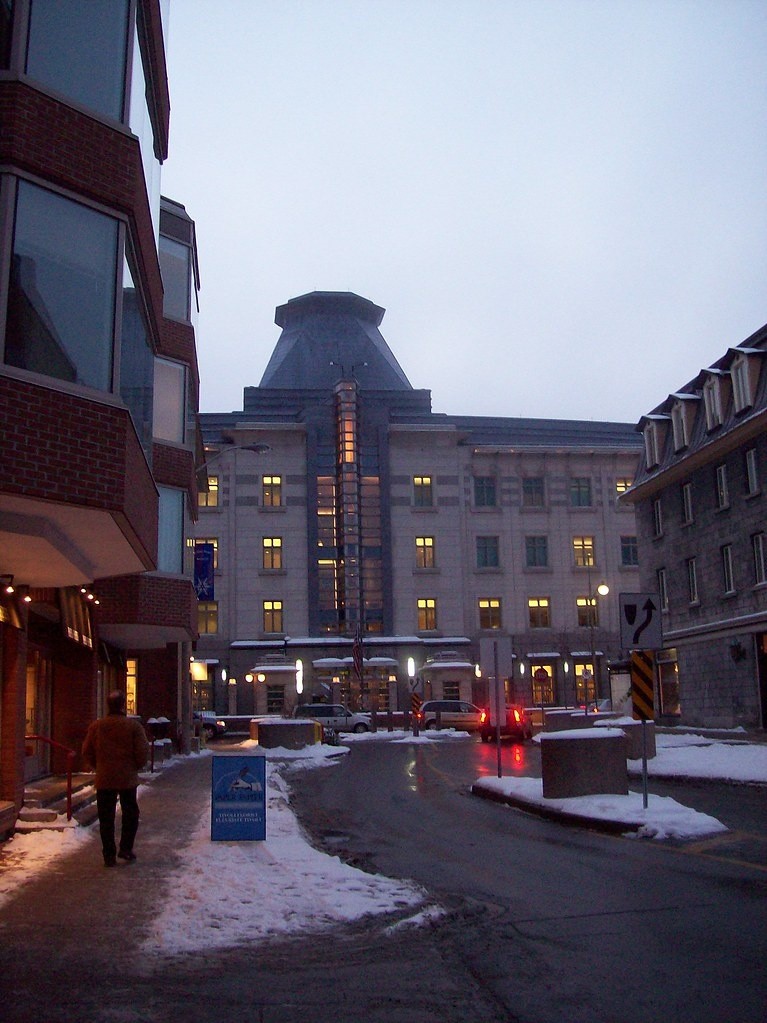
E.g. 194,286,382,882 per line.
82,691,149,868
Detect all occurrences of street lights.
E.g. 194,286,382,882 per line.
588,583,610,712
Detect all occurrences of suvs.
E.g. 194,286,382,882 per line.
292,702,373,734
418,700,486,736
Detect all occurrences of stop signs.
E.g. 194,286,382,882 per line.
534,667,548,683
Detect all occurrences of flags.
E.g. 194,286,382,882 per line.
352,627,361,681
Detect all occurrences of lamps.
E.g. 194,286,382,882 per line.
0,574,15,592
81,584,87,593
20,583,31,601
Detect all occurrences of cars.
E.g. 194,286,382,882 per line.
193,712,227,742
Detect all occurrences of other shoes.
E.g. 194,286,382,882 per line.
105,860,117,865
117,849,137,860
